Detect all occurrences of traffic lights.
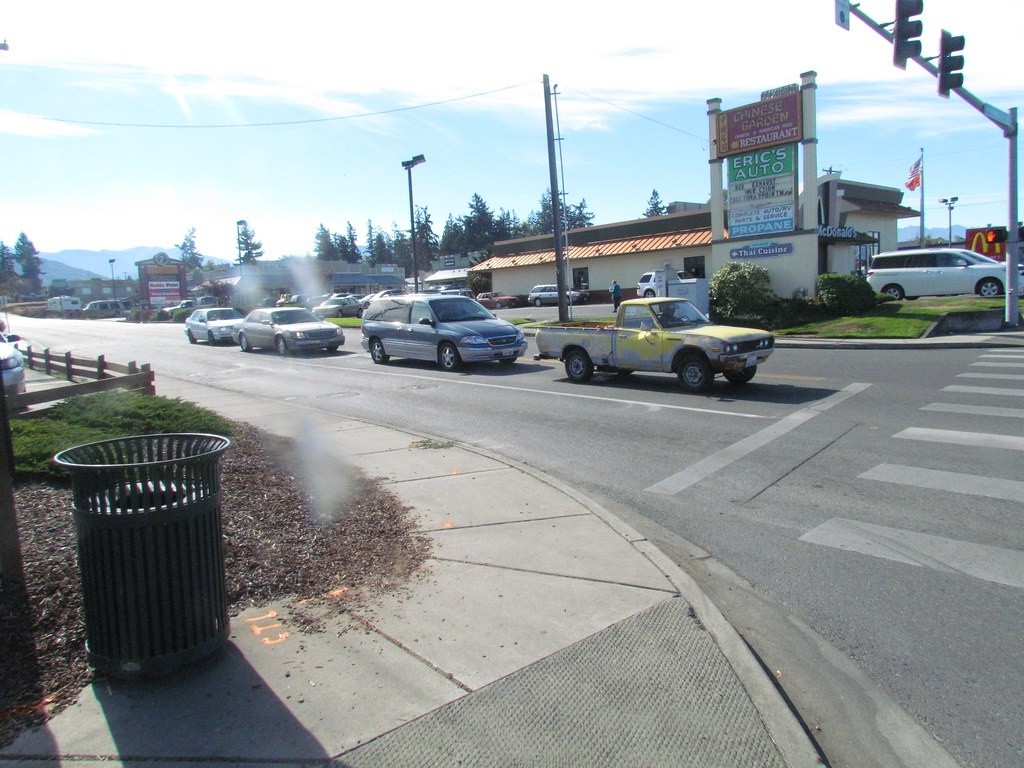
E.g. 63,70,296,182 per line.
892,0,924,70
986,225,1008,244
938,29,966,99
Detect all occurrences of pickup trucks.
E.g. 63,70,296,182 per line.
636,268,703,299
532,297,775,397
162,295,218,319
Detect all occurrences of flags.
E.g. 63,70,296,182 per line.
905,157,920,190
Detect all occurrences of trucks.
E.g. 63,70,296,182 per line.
48,296,81,312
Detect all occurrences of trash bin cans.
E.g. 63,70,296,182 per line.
52,433,231,684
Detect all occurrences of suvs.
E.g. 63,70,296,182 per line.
527,284,589,307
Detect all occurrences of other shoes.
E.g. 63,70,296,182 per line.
612,311,617,313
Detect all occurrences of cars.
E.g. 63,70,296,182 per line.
187,306,244,345
476,291,520,310
0,331,27,397
233,307,346,355
278,283,474,320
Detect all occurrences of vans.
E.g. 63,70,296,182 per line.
866,244,1024,300
83,300,125,312
360,292,533,370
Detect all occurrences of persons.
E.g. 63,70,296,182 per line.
659,304,682,327
609,280,622,313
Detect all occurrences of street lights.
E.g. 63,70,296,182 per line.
400,153,428,294
235,220,247,305
109,258,118,301
937,196,959,249
